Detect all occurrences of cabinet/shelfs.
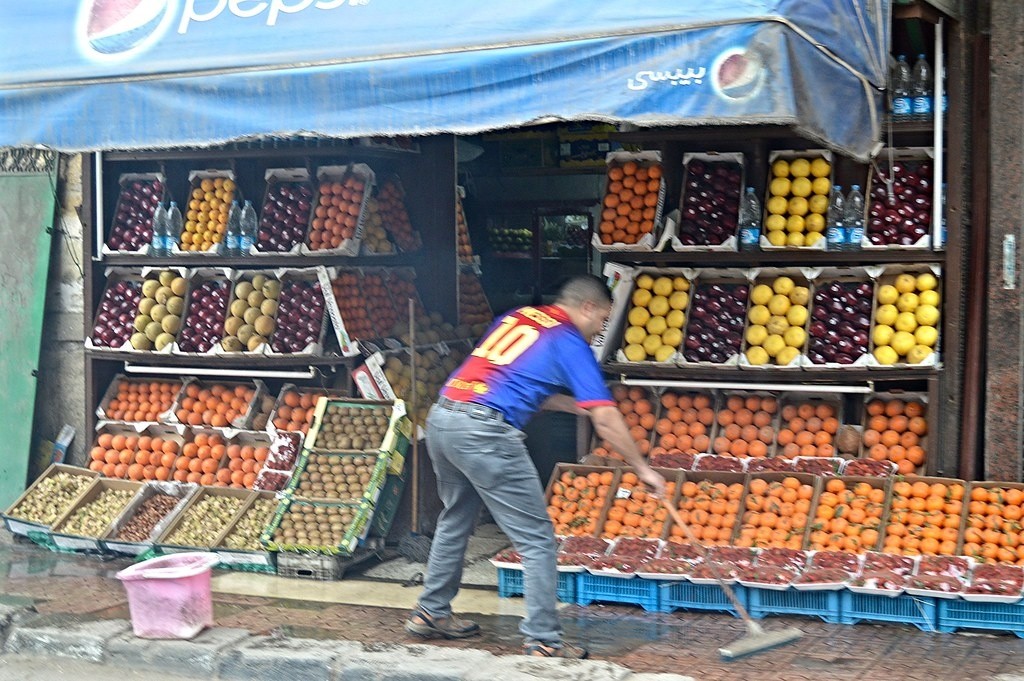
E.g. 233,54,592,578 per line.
86,139,440,549
475,208,593,318
601,114,947,481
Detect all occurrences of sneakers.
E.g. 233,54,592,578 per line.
405,605,480,640
522,638,588,660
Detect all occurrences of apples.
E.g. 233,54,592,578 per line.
91,178,326,353
867,160,933,246
307,176,498,432
678,159,740,247
765,158,831,248
871,272,940,365
624,275,871,364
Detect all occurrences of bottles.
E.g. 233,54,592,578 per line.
166,201,183,258
826,186,845,251
939,183,947,246
226,200,241,258
741,187,761,251
892,55,912,122
239,200,258,259
843,185,864,252
912,54,933,121
942,65,948,113
151,201,167,258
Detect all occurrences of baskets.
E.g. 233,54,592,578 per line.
277,544,379,581
498,540,1024,640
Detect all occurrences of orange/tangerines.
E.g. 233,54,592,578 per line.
542,385,1024,567
89,379,339,490
487,226,533,253
600,160,660,244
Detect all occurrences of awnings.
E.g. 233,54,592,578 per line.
1,0,891,162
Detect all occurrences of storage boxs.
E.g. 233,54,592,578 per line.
1,171,499,583
489,136,1024,640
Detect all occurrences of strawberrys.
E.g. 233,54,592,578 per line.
492,536,1024,597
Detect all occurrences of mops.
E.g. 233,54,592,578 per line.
654,488,799,661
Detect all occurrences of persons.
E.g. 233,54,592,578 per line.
403,274,666,658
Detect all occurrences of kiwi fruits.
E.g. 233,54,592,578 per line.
270,405,392,547
